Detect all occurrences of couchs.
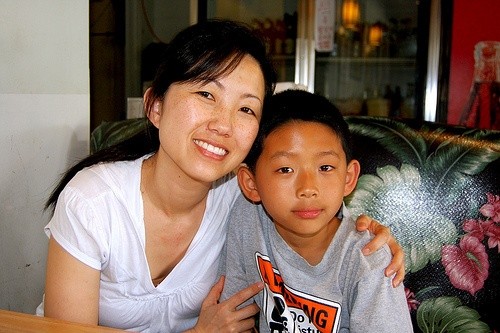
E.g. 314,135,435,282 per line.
89,116,500,333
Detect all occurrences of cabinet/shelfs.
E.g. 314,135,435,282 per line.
189,0,441,123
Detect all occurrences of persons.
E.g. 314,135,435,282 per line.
211,89,413,332
36,18,405,333
